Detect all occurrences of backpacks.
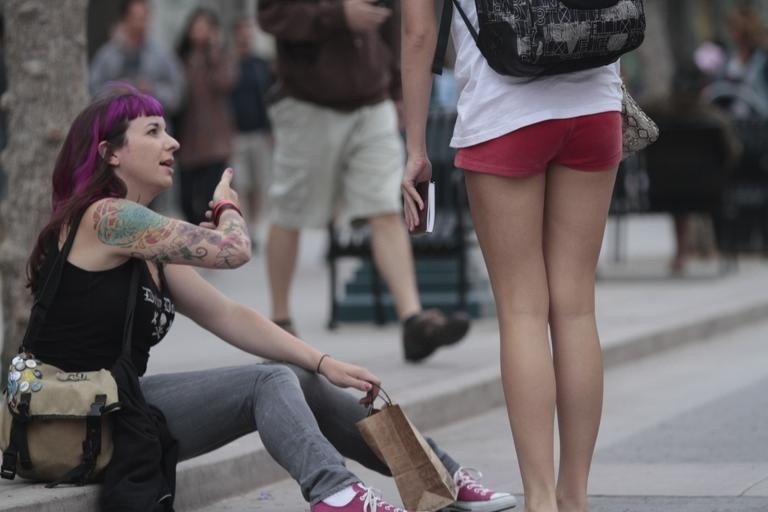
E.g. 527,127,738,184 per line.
454,1,645,78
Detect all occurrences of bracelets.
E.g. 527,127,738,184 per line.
318,354,329,373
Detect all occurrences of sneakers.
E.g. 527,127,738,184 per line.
310,482,405,511
441,466,517,511
402,305,469,362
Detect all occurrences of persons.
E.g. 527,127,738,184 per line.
83,0,768,272
399,0,622,510
255,2,470,364
20,83,517,511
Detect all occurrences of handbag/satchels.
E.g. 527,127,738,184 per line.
621,84,660,162
356,401,459,511
0,351,122,482
